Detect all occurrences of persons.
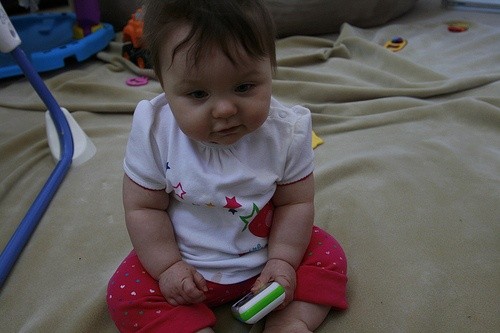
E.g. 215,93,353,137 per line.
105,0,348,332
